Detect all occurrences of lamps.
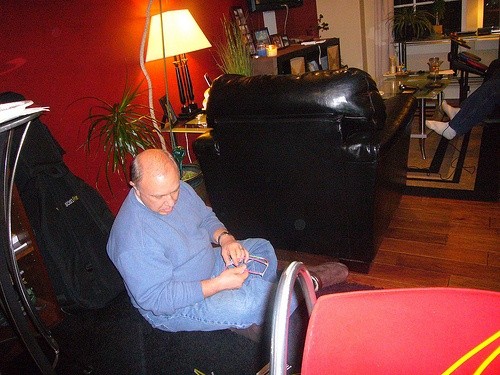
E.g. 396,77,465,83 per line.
145,7,212,121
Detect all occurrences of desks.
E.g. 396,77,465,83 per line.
252,36,340,75
376,69,453,159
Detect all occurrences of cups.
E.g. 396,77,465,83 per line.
429,57,439,72
390,56,396,73
397,66,402,72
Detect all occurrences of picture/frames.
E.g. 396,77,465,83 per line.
281,33,290,47
270,33,285,49
252,26,272,46
159,93,179,129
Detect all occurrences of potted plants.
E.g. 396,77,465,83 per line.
304,14,329,41
430,0,446,40
385,5,437,42
64,57,205,202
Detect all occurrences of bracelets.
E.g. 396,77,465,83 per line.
218,233,230,246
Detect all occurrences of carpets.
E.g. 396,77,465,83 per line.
405,109,500,203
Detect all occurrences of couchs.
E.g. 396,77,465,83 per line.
191,73,418,274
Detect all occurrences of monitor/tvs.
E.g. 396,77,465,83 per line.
248,0,304,13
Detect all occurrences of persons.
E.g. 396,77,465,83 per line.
424,58,500,140
105,149,349,333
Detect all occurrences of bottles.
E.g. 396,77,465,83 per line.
257,40,265,56
